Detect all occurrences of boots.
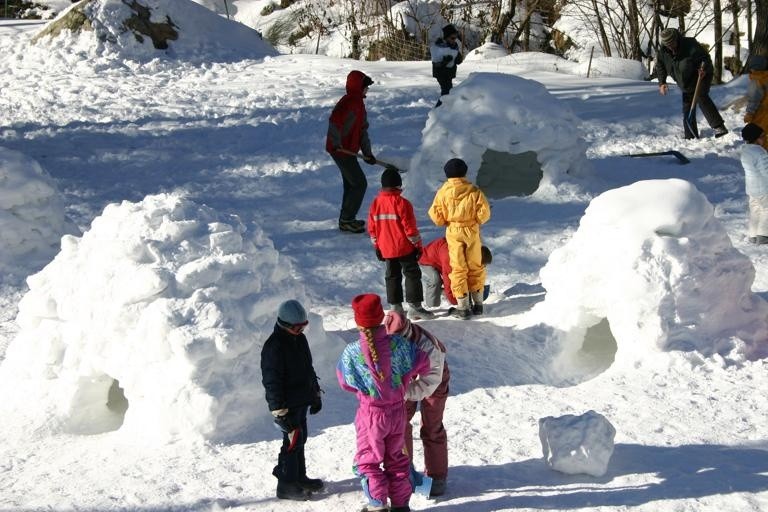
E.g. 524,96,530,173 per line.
390,303,407,316
276,464,312,501
407,302,434,320
299,458,323,489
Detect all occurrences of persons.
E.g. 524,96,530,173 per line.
327,71,377,233
336,293,431,511
260,299,323,502
416,234,492,313
655,27,728,138
741,122,768,245
384,311,449,496
368,166,434,321
428,158,491,320
743,54,768,153
430,24,462,108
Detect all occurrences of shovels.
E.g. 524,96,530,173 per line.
686,60,706,138
620,151,691,165
337,149,408,173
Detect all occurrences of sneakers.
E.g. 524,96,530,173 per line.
339,221,365,233
471,305,482,315
354,220,365,225
448,307,470,319
431,479,447,496
714,123,727,138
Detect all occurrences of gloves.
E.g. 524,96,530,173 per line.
375,249,385,262
743,112,753,122
415,247,423,260
364,155,376,164
309,397,321,414
273,416,293,433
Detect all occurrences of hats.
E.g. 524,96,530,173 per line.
381,167,401,186
361,72,373,87
443,158,467,177
386,311,409,335
351,294,384,328
749,55,766,70
277,299,307,330
441,24,458,40
659,27,677,46
740,123,762,143
